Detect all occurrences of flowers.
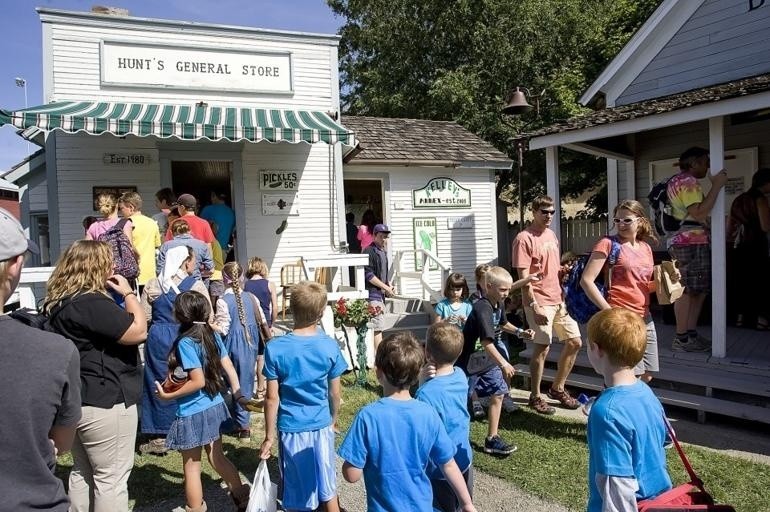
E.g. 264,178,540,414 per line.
334,292,382,324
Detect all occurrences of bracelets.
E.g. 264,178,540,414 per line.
515,327,525,339
121,291,138,302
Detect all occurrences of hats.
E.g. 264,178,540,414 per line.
679,146,709,162
211,185,224,192
373,223,391,234
177,194,197,208
0,207,40,262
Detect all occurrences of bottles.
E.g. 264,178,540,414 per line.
162,366,191,394
578,392,597,415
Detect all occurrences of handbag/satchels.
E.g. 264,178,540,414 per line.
257,322,272,355
637,478,736,512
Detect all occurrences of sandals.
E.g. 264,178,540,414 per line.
735,320,744,327
756,322,770,331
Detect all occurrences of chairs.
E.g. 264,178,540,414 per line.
279,264,308,322
314,264,339,295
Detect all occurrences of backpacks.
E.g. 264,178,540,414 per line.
98,226,140,281
8,307,55,333
565,235,621,325
647,183,681,236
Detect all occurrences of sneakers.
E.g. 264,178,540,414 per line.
546,385,578,409
671,329,712,353
472,396,491,418
171,499,208,512
503,397,519,413
237,429,251,443
138,440,171,454
484,433,517,455
254,388,267,399
529,393,556,414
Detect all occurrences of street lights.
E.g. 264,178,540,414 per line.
14,76,31,156
499,85,547,234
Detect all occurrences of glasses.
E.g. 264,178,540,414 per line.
538,209,555,215
612,218,640,225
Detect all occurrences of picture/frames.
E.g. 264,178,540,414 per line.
92,184,138,213
413,215,440,272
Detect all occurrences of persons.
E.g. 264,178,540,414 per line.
431,262,544,456
363,223,399,368
662,145,729,354
584,307,673,512
84,187,280,454
154,290,264,511
355,209,379,254
414,323,473,512
257,279,349,512
42,239,149,511
559,251,599,323
346,212,362,288
576,197,684,400
723,165,770,332
337,332,476,512
508,193,584,417
0,207,82,511
